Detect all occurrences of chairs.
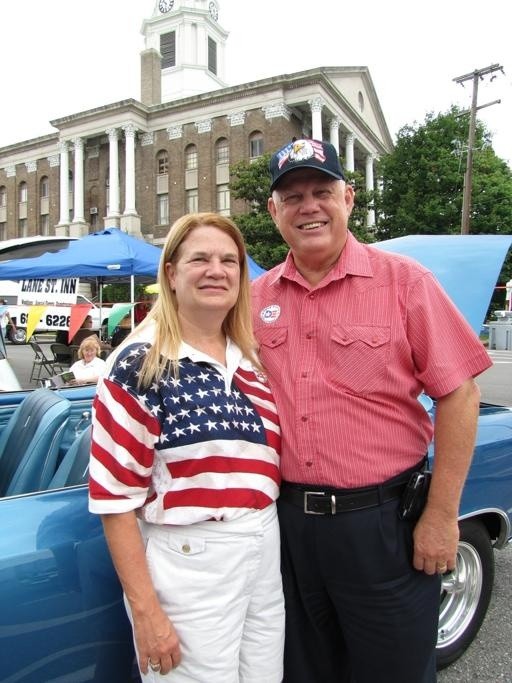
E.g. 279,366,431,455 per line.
28,341,57,384
0,388,72,497
50,343,73,377
48,424,91,490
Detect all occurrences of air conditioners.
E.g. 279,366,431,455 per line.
90,207,99,214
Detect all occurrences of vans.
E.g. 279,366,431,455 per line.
1,234,512,683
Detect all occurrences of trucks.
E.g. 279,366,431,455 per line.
0,294,112,345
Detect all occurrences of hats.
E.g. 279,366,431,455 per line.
269,138,346,192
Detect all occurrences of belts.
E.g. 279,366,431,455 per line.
280,461,427,515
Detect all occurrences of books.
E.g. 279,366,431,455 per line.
52,372,75,387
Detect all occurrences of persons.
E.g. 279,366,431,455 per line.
89,212,286,683
67,315,131,386
250,139,493,682
0,300,17,359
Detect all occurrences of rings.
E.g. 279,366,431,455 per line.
150,661,161,669
438,566,447,570
